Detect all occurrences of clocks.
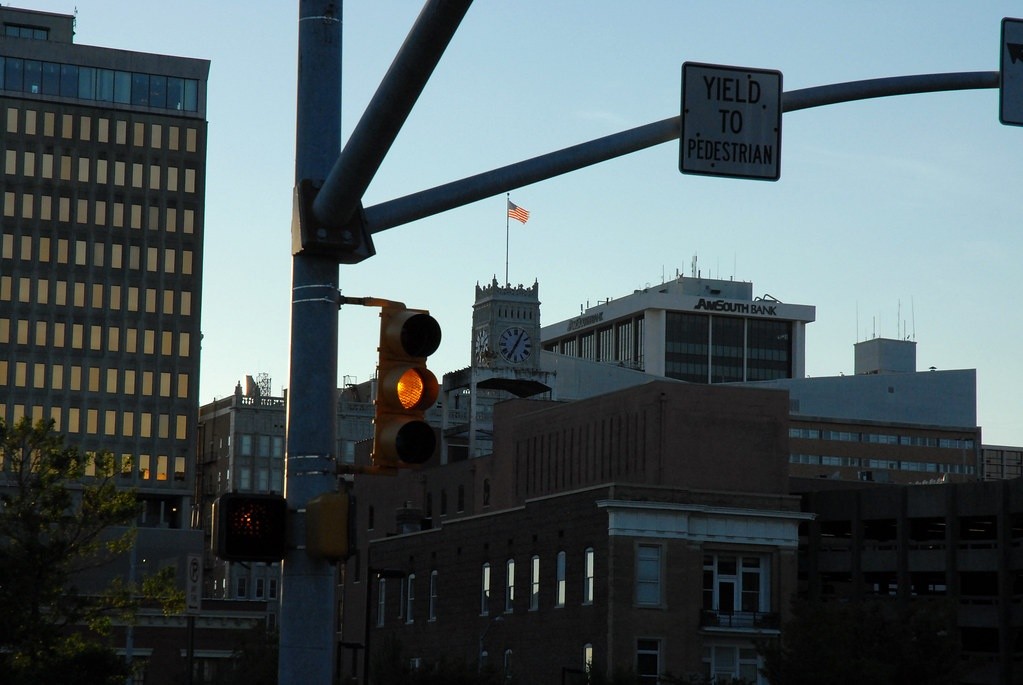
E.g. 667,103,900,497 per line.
498,326,533,366
474,330,491,368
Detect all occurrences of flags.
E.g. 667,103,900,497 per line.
508,201,529,224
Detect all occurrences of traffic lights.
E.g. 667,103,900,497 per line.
372,304,442,476
